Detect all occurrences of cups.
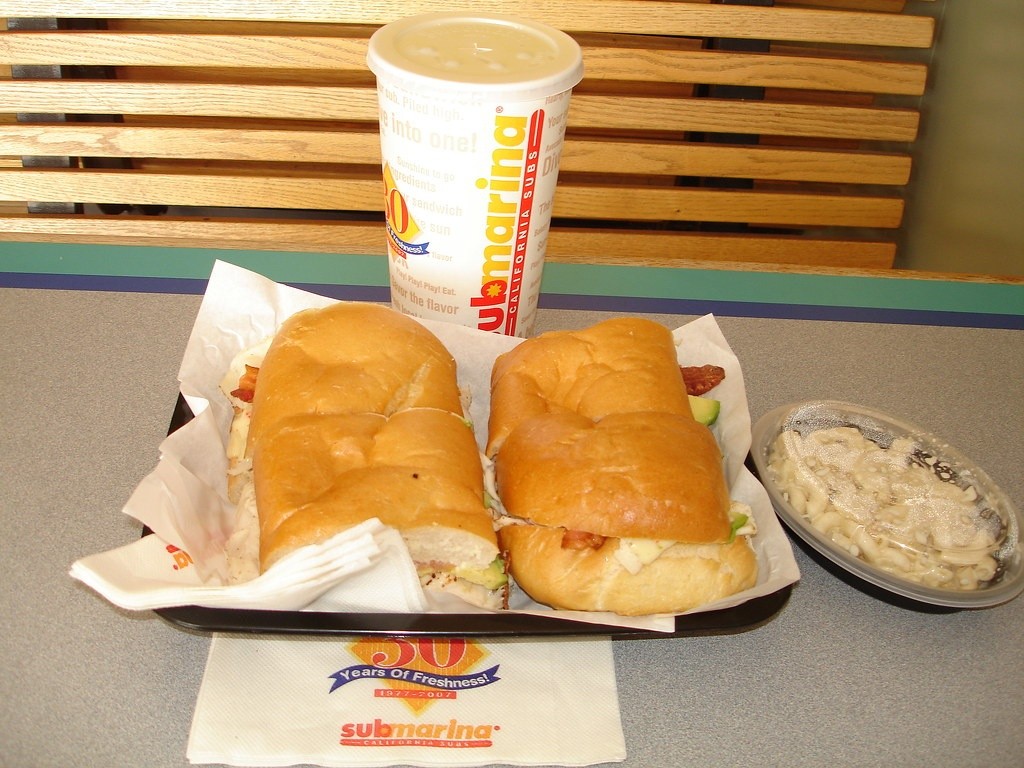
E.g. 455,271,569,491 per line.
364,12,584,338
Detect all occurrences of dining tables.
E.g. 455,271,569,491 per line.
0,241,1024,768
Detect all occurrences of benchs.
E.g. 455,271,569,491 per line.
0,0,933,275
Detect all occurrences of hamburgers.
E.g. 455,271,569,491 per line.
220,302,760,617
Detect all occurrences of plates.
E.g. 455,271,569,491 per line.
748,399,1024,611
